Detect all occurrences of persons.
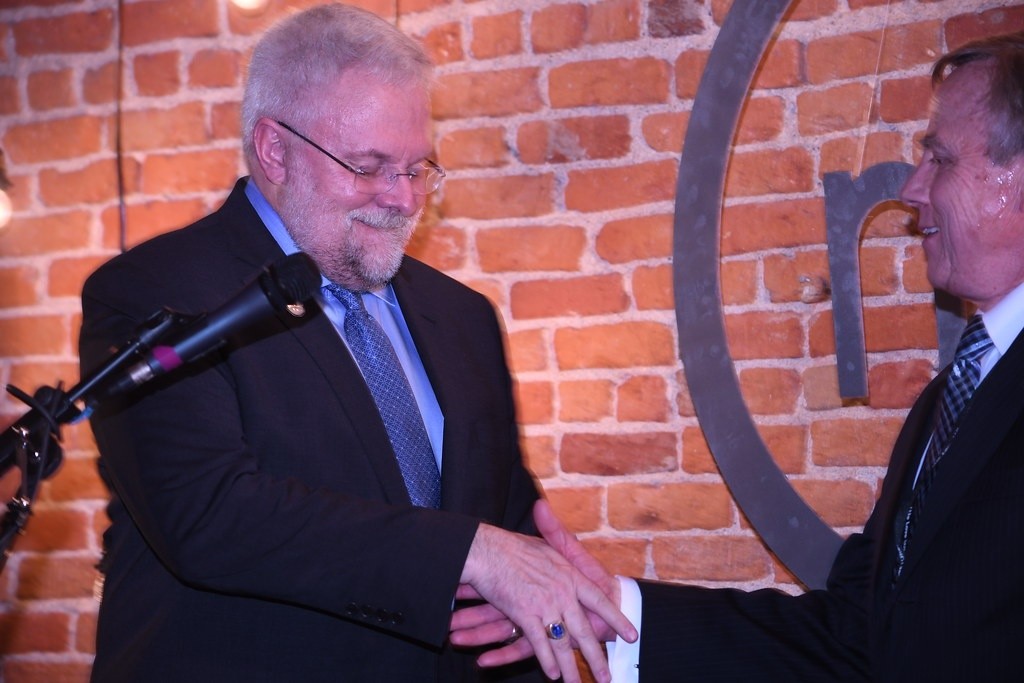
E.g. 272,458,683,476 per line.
446,33,1024,683
78,2,639,683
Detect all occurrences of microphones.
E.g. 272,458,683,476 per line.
105,252,322,399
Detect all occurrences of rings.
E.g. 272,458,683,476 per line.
507,624,524,642
545,620,565,640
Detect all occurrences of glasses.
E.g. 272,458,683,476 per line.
278,120,446,195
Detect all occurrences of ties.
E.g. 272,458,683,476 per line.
901,314,996,577
327,285,444,509
